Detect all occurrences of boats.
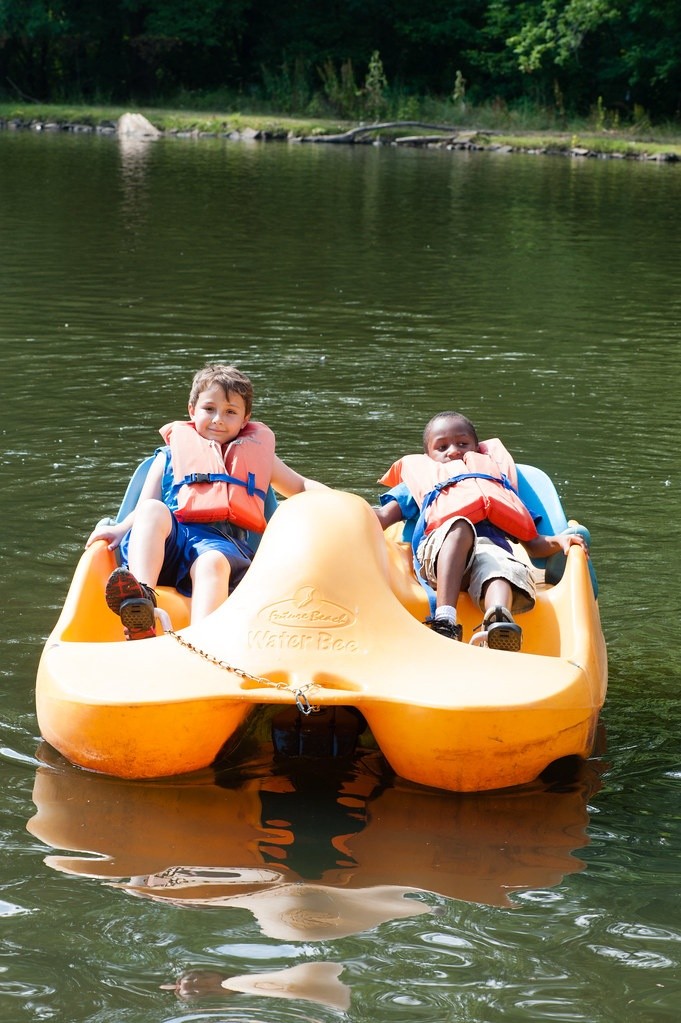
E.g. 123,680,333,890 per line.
33,450,611,794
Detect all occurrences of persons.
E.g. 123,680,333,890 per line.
84,364,333,640
373,411,588,651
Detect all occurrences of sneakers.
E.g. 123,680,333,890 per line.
430,617,463,642
105,566,156,616
481,605,515,649
